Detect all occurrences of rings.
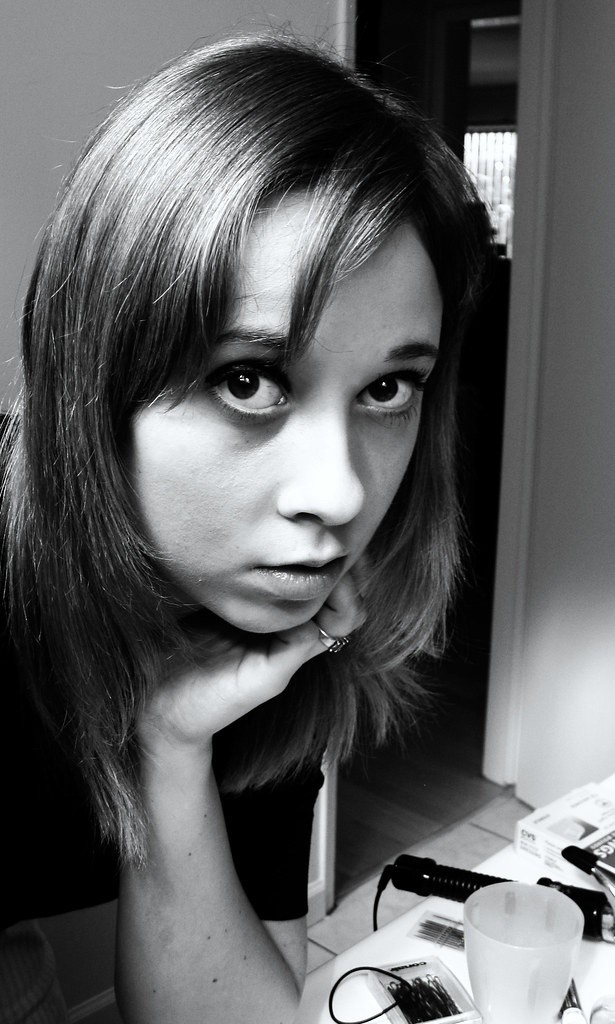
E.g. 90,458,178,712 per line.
314,618,349,653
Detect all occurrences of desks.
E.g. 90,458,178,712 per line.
304,776,615,1024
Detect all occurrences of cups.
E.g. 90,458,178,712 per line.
463,881,585,1024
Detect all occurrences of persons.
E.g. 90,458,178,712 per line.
1,34,500,1023
